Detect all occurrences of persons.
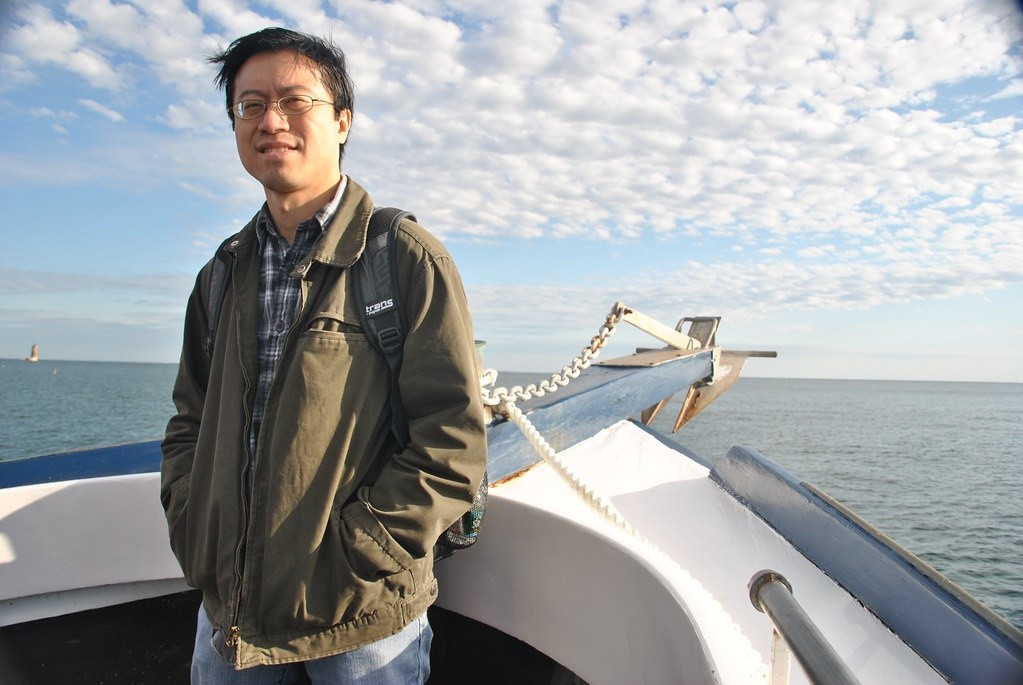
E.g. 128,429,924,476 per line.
160,28,489,685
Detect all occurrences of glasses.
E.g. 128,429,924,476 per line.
227,95,350,123
